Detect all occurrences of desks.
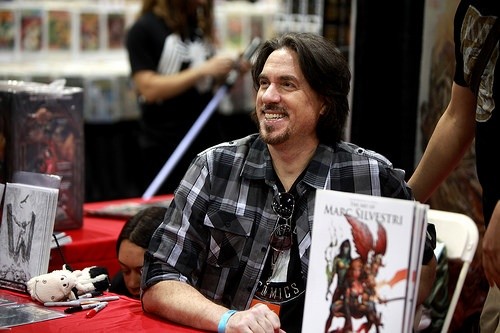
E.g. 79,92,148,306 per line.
0,193,211,333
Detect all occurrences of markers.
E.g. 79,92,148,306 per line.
64,303,101,314
66,292,120,305
85,302,107,319
44,301,81,307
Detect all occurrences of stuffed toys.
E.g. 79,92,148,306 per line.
26,264,108,304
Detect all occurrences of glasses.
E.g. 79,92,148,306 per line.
270,192,294,250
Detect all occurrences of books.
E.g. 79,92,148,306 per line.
0,182,59,295
301,188,429,333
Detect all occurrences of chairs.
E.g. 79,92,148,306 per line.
420,209,479,333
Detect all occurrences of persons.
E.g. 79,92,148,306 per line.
406,0,499,332
110,206,168,298
124,1,254,193
140,31,437,333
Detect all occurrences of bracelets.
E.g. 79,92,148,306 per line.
218,309,234,333
